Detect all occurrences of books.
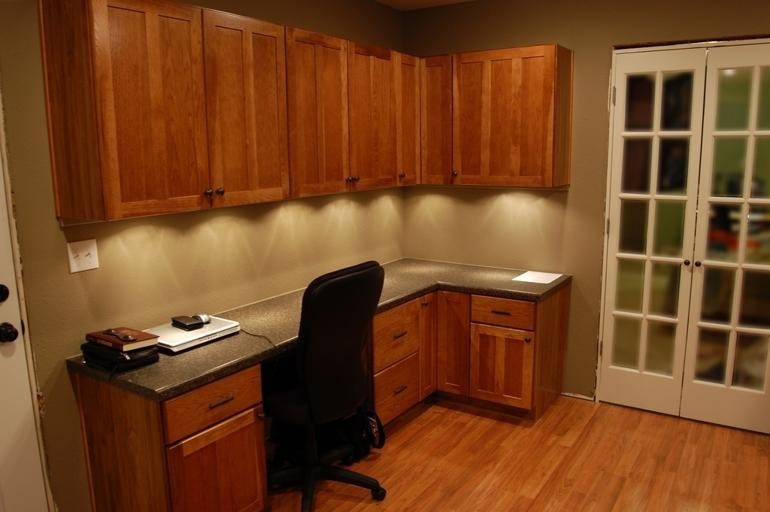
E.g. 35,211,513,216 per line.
85,325,160,351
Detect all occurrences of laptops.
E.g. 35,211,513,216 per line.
141,314,241,354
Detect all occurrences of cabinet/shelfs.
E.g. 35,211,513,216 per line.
285,24,394,200
38,0,290,223
72,363,265,512
488,43,573,188
394,51,422,187
422,50,488,186
372,294,433,427
437,283,572,422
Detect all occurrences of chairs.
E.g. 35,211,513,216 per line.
265,261,385,511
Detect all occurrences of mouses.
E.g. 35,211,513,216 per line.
192,312,211,323
